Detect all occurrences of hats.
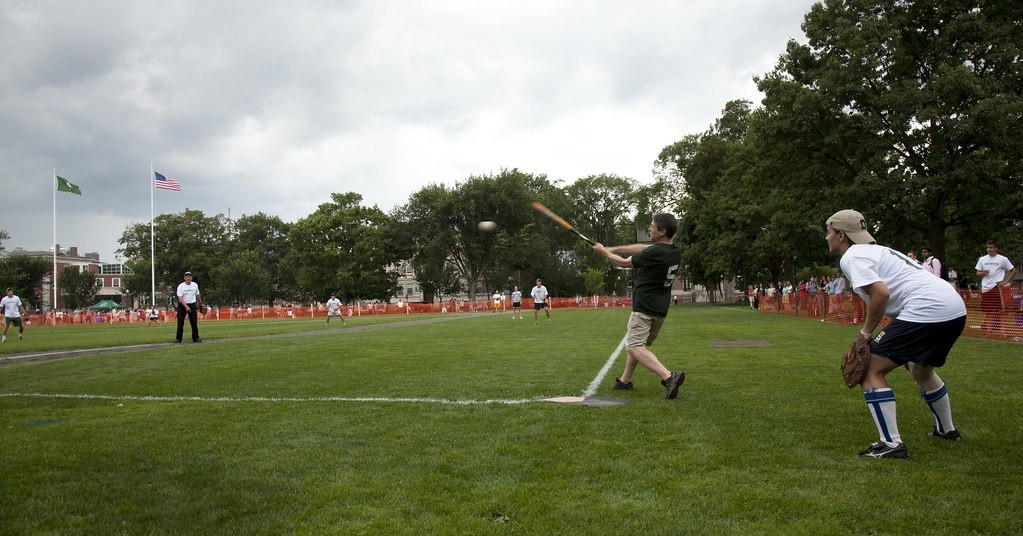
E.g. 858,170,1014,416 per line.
826,209,876,244
184,272,192,275
6,287,12,293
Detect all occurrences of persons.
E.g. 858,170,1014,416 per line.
591,213,685,400
826,208,967,457
174,272,205,343
907,246,958,289
325,293,346,326
531,279,551,326
41,304,252,326
674,294,678,305
277,302,296,318
439,288,501,313
574,294,583,308
0,287,27,342
975,241,1016,330
748,272,846,315
397,299,404,313
366,301,380,316
511,286,523,319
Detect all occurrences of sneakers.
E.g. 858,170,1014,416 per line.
661,371,685,400
609,377,634,391
928,425,963,441
858,442,907,459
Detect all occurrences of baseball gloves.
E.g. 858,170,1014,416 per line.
334,307,338,311
838,333,871,390
23,314,29,321
199,303,207,316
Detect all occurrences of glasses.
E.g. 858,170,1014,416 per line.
921,250,928,253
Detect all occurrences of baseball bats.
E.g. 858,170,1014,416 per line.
531,201,597,246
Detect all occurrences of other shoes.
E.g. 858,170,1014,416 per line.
18,335,22,340
513,317,515,320
197,339,201,342
520,317,522,319
547,319,552,325
2,338,6,342
175,341,181,343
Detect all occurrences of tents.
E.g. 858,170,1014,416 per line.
88,299,126,310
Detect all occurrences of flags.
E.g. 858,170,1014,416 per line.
154,171,181,191
56,175,82,195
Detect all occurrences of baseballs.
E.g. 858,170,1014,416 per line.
484,221,496,232
